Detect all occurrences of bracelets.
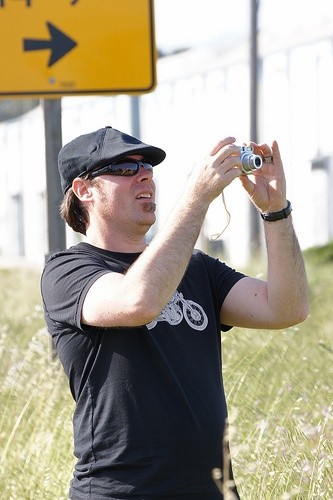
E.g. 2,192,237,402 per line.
261,200,292,221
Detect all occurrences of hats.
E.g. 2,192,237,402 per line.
57,126,166,195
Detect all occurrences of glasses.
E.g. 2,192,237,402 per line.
87,157,152,180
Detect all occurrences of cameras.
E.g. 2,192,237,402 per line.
228,145,263,178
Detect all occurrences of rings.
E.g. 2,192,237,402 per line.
263,156,273,163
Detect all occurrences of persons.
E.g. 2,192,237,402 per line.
41,126,310,500
